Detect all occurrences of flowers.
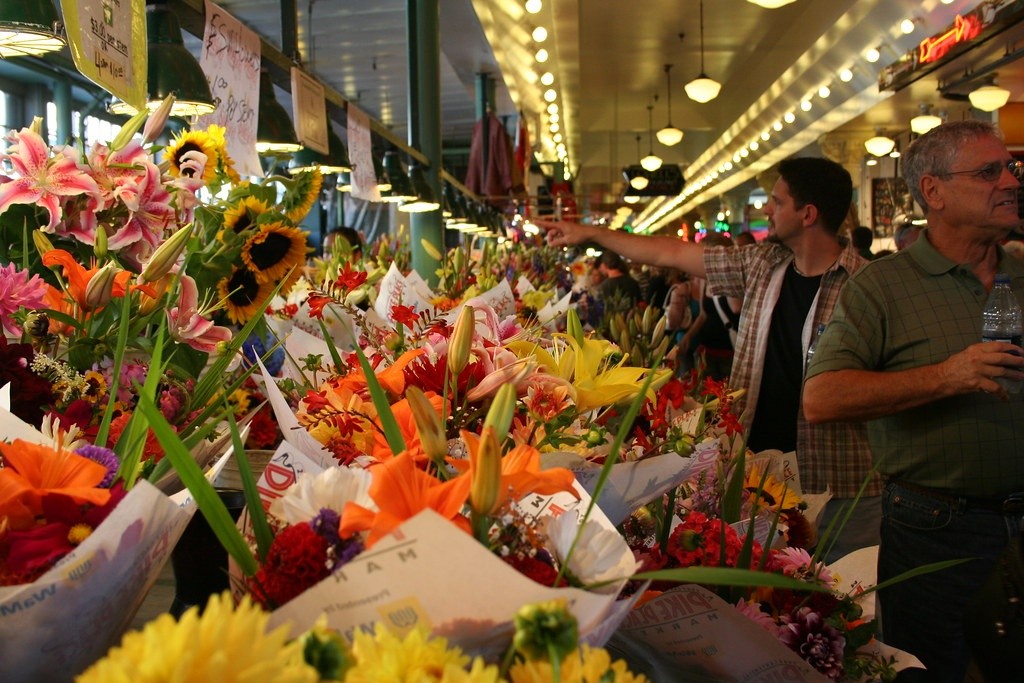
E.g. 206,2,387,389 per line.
144,121,322,374
0,91,215,432
0,232,989,683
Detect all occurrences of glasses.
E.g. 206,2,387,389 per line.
933,159,1024,182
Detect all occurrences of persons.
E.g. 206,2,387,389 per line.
592,232,755,424
802,120,1024,683
852,223,925,261
530,157,883,637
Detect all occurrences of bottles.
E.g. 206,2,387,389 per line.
981,273,1024,395
806,324,826,371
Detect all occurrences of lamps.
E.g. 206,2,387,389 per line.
397,162,441,213
682,0,722,104
286,103,357,176
655,64,684,148
864,127,896,158
370,152,419,203
442,179,498,237
640,104,664,172
105,0,216,117
630,134,649,190
335,142,393,192
890,140,901,159
0,0,68,58
865,155,878,166
910,103,942,135
256,68,305,155
969,72,1011,113
